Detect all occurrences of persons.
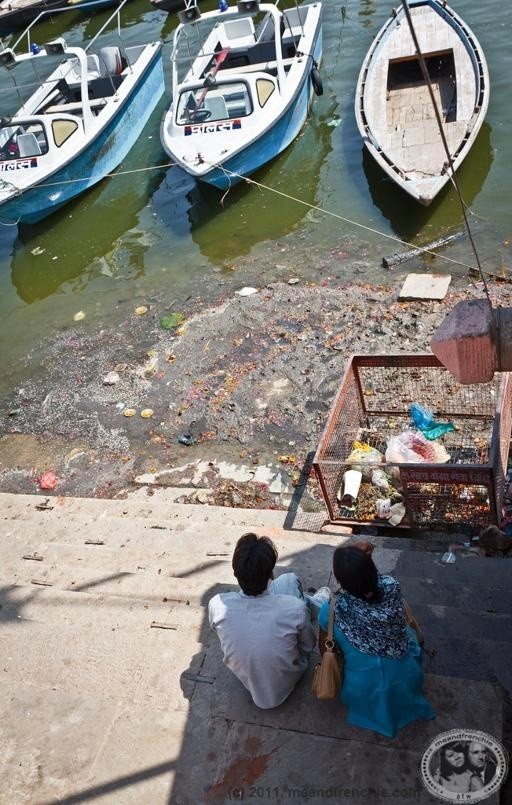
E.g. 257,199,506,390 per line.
432,742,486,794
468,738,496,786
206,532,332,709
316,542,438,738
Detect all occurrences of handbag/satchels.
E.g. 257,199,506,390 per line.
311,598,344,704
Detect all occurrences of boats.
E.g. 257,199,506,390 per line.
0,0,197,28
12,89,174,306
361,116,490,261
184,60,333,265
0,36,166,226
354,1,491,207
159,0,323,193
7,1,217,47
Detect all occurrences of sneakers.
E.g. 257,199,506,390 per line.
312,585,332,606
